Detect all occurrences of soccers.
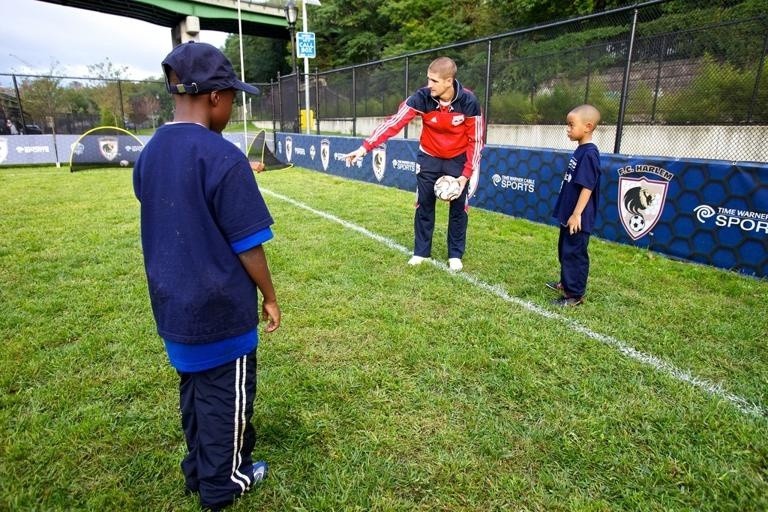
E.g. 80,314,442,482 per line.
628,215,645,232
434,175,461,201
376,168,381,175
120,159,129,167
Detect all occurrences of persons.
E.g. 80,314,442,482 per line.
545,103,603,308
132,40,283,512
1,117,27,135
338,55,485,272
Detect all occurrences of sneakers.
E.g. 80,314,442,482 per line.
449,258,463,271
408,255,432,266
252,460,268,484
546,279,565,292
552,294,583,307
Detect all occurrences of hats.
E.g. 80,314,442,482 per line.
160,40,260,96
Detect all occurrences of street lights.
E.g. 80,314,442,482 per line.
281,0,306,136
